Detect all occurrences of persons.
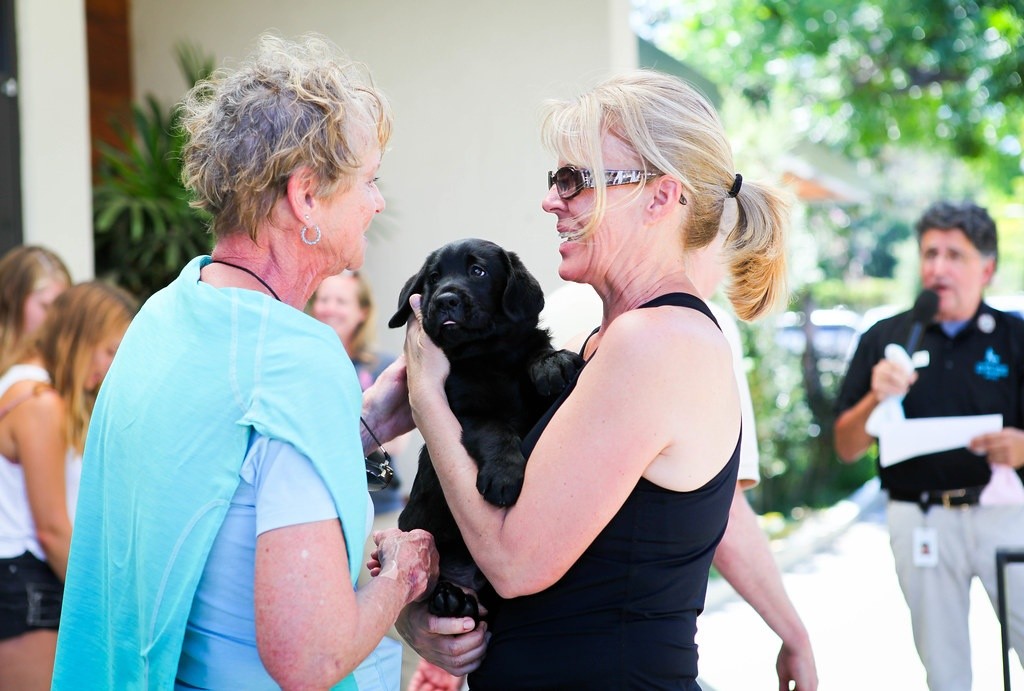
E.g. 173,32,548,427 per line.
922,544,929,553
830,200,1024,691
0,42,818,691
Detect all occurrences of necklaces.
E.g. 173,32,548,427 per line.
213,259,282,302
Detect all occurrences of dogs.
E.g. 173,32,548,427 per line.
385,237,587,634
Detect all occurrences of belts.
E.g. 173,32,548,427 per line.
930,487,986,510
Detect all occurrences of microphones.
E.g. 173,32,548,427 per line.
904,288,939,358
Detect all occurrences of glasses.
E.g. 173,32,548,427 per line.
545,164,689,208
360,417,396,492
